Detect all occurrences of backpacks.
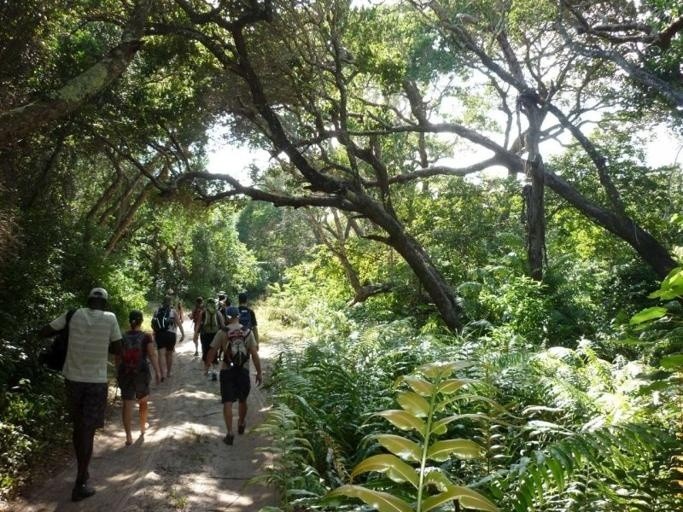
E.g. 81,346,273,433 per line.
120,332,142,375
150,308,169,332
201,307,219,334
221,325,250,367
240,308,252,328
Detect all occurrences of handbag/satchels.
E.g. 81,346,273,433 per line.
46,309,77,372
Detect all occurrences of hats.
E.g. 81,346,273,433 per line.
87,287,108,300
226,306,240,316
195,291,248,304
128,310,144,321
161,288,175,306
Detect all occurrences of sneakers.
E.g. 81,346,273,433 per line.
236,416,247,434
160,351,218,384
222,434,235,444
141,421,150,434
76,471,90,482
124,439,133,446
71,487,95,500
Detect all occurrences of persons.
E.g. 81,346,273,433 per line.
188,296,203,357
191,299,225,381
36,288,123,503
148,293,185,382
203,307,262,445
235,293,260,353
215,291,231,320
113,311,161,446
